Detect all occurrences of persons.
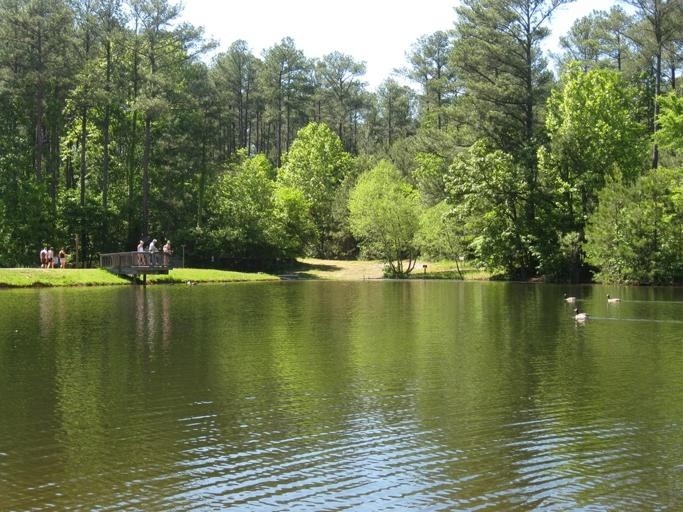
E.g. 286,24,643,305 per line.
39,247,48,269
46,246,55,269
147,238,158,267
136,240,146,266
57,247,65,269
162,240,173,268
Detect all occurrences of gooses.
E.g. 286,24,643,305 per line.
563,292,577,303
572,307,589,323
606,293,621,304
